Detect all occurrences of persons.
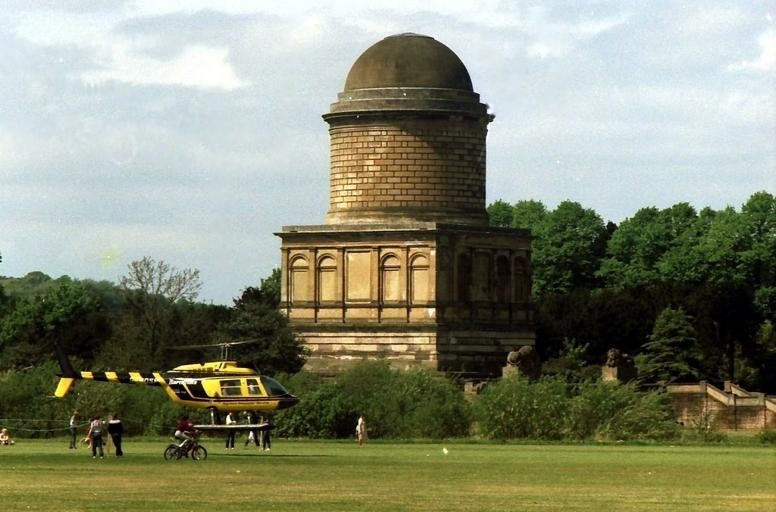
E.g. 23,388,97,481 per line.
106,411,124,457
174,414,197,449
258,412,271,452
0,428,8,445
86,418,94,449
243,411,260,448
355,413,368,446
225,410,236,449
86,414,104,459
68,410,79,449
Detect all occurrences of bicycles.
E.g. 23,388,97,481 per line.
164,430,207,461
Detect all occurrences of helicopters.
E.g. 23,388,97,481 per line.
54,339,300,429
